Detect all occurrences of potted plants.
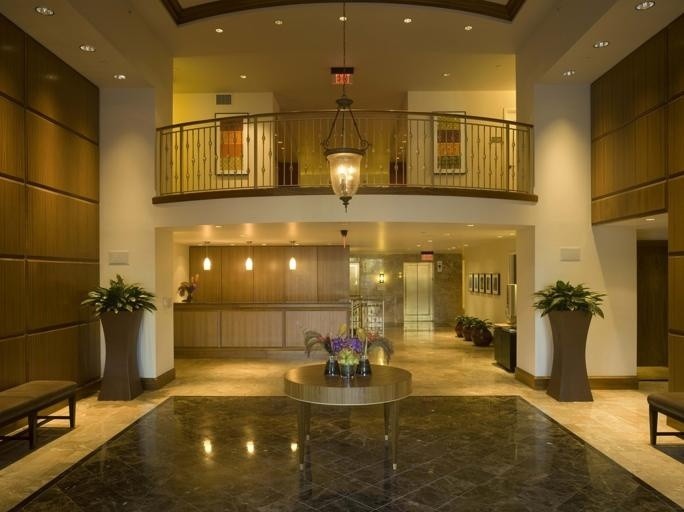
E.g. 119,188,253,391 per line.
471,318,492,346
528,276,612,401
81,274,155,400
461,316,474,341
454,315,464,336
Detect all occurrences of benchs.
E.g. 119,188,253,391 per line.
1,379,78,461
647,391,684,450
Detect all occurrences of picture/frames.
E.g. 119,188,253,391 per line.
431,110,468,175
467,273,500,294
214,112,249,176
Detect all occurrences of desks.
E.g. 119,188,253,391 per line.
284,363,412,471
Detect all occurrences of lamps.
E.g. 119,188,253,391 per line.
244,240,253,270
203,243,212,271
320,2,370,212
288,240,296,270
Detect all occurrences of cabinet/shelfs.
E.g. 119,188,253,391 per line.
492,322,515,371
